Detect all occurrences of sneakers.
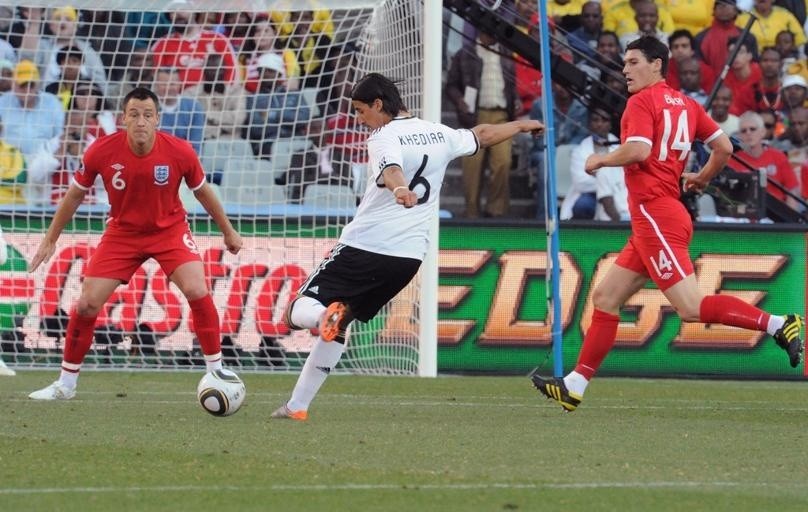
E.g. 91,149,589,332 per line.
29,381,74,403
270,405,307,422
531,371,582,410
318,301,345,344
774,312,803,364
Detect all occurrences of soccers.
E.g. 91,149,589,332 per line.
198,368,245,415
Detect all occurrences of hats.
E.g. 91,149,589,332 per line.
255,55,284,81
12,60,39,87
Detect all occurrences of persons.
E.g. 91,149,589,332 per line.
269,71,546,421
21,87,245,401
446,1,806,222
529,35,807,414
3,1,370,208
